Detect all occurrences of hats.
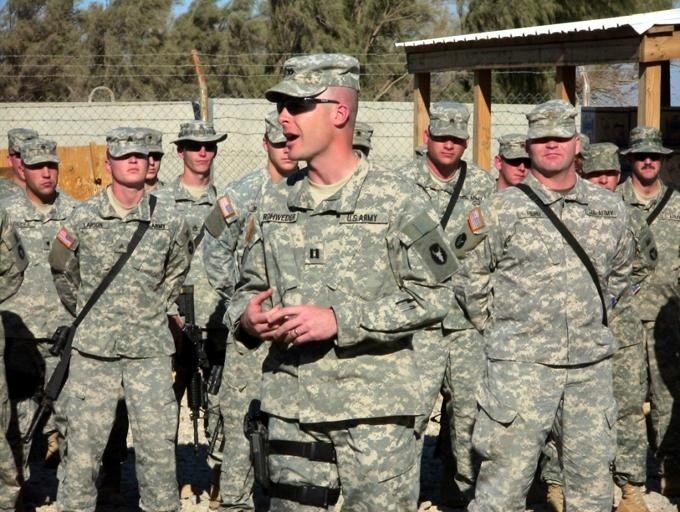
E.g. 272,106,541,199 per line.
496,132,530,160
170,120,227,145
582,141,621,176
428,100,471,140
106,128,164,157
619,126,675,157
265,111,286,144
526,100,577,140
353,121,375,148
6,128,61,165
262,53,362,101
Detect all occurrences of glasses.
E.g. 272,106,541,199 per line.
276,97,340,115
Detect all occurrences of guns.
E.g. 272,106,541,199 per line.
176,284,224,458
250,432,270,511
23,325,73,443
